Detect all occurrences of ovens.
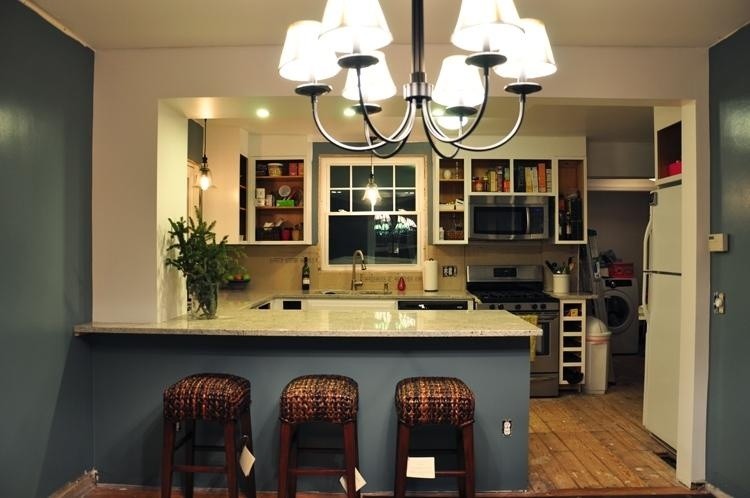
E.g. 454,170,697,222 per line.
509,310,559,374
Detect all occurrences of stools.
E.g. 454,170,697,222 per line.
277,374,361,498
394,376,476,498
161,373,256,498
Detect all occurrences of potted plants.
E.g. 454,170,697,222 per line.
592,249,616,278
162,203,249,321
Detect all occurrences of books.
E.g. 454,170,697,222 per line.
473,160,552,191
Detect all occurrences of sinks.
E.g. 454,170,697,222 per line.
354,290,393,295
323,290,352,295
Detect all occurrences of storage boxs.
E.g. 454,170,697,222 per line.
609,262,633,278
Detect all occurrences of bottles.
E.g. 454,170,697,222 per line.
559,209,572,240
301,256,311,290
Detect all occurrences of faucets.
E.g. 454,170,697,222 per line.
351,250,367,290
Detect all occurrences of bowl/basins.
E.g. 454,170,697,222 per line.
226,277,250,290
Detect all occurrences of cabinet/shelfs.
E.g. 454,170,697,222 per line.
555,137,588,246
201,127,252,245
558,300,587,394
426,138,471,244
472,159,556,197
249,135,318,245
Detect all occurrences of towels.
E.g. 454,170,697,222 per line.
517,315,539,363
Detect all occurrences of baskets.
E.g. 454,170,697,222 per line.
445,230,464,239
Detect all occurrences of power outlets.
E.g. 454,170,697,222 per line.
442,265,458,278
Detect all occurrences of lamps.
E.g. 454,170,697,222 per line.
193,119,216,191
278,0,558,160
361,137,381,214
373,188,417,256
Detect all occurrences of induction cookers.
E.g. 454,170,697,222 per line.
469,289,559,301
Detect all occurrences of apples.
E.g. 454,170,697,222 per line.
226,274,250,281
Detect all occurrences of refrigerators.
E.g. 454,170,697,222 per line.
640,185,681,458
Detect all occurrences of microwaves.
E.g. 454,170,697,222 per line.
469,195,550,240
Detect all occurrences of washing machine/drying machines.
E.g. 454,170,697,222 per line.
602,276,639,355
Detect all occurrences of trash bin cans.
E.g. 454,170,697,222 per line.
581,315,612,394
